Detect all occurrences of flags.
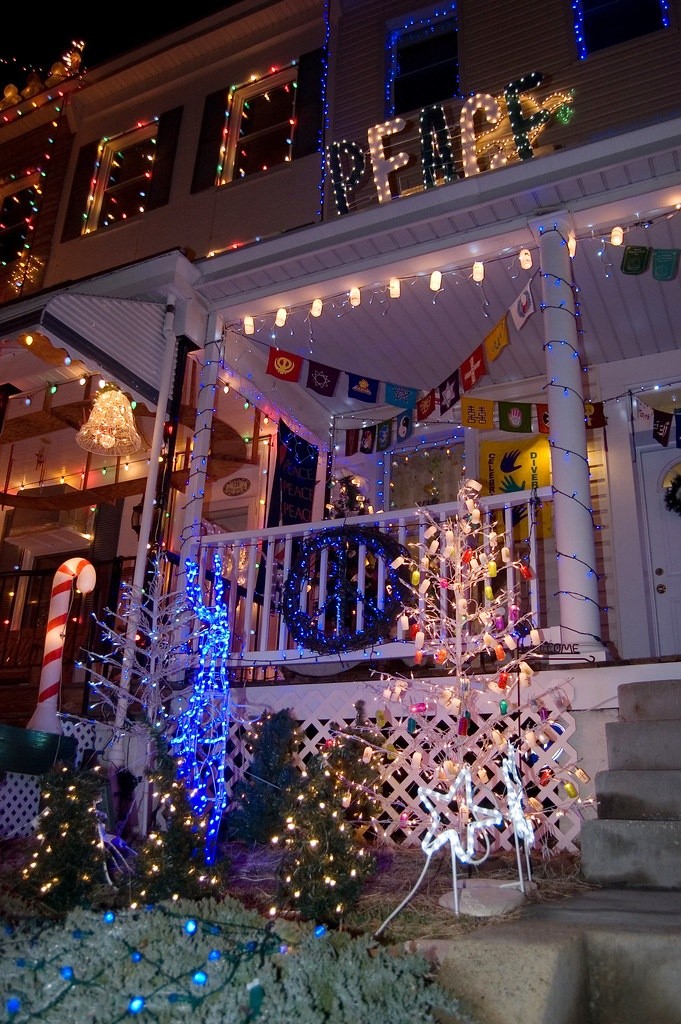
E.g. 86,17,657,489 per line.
397,406,413,444
265,346,302,383
485,313,508,364
584,397,606,429
509,277,535,331
438,367,460,417
460,343,487,394
384,383,417,409
478,434,552,543
345,428,359,456
536,404,550,434
348,372,380,403
306,361,341,397
416,387,435,423
461,396,496,430
359,424,377,455
375,418,392,452
498,402,532,433
635,397,681,449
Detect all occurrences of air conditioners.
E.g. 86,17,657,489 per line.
5,483,97,556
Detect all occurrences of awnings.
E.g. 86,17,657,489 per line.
0,295,177,422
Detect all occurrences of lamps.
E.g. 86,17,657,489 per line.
75,386,141,456
131,493,144,542
